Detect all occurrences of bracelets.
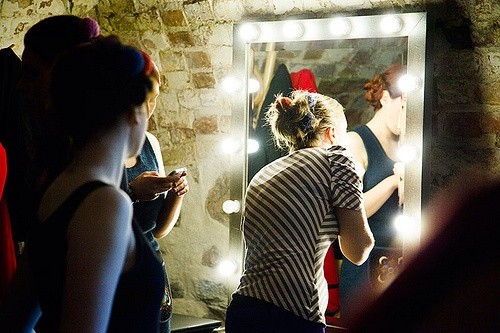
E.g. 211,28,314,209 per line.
128,182,139,203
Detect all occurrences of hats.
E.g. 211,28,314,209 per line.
22,14,99,78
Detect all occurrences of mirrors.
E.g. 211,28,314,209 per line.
227,11,427,304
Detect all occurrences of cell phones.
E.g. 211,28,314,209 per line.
169,168,186,176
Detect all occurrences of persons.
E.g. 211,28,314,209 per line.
224,93,374,333
338,64,404,328
0,16,190,333
